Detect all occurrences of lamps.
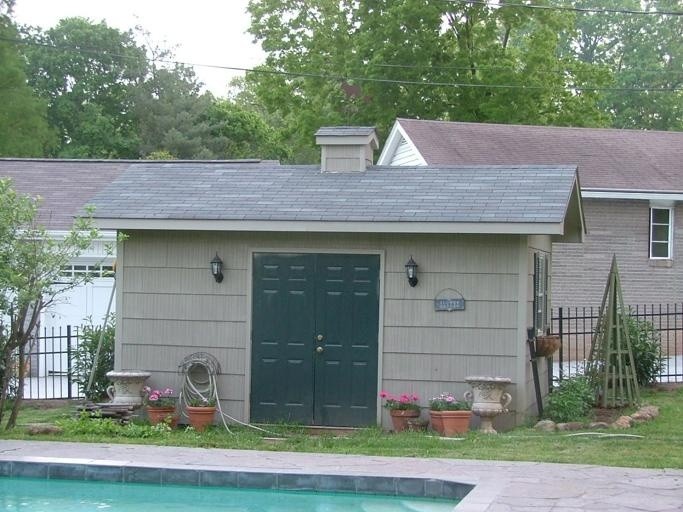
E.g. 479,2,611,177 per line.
406,255,420,287
210,250,224,283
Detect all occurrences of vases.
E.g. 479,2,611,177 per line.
147,406,176,429
392,405,471,437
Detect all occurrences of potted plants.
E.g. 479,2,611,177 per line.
184,397,216,433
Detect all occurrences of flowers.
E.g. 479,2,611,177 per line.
378,389,472,411
141,383,178,409
